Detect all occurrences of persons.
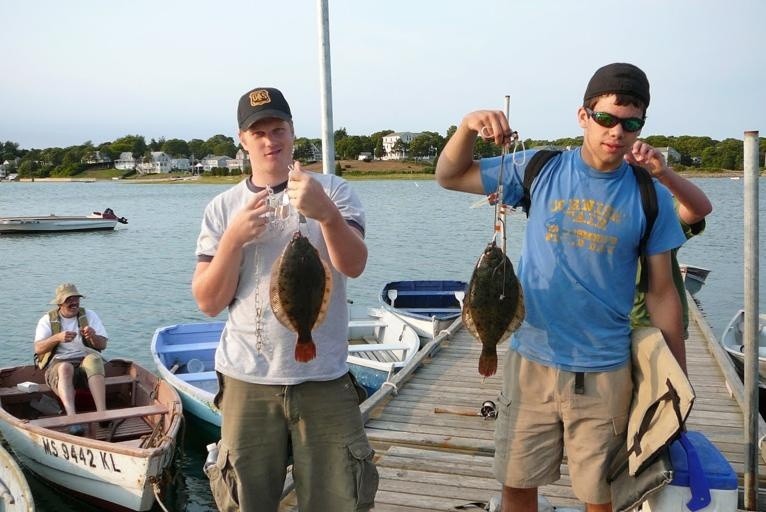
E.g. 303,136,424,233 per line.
189,86,380,512
621,138,713,340
434,61,689,511
32,280,114,436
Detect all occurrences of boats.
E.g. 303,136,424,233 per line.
345,304,421,398
150,320,227,428
151,322,244,427
676,265,711,295
378,281,473,339
377,280,470,348
680,265,710,296
320,303,420,389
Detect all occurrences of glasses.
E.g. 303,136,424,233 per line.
585,107,643,133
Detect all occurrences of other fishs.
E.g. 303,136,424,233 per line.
462,241,526,384
268,229,332,365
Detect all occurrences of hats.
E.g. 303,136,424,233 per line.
48,283,85,306
583,62,650,107
239,87,292,133
